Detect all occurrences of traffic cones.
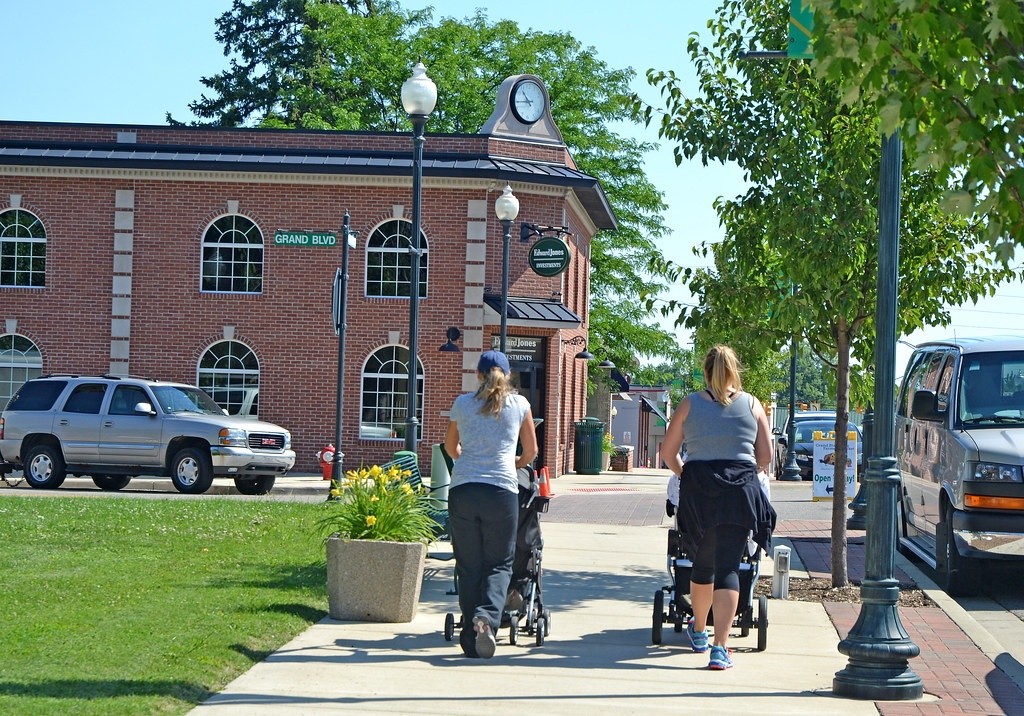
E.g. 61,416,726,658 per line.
537,468,553,499
533,470,539,496
543,465,556,496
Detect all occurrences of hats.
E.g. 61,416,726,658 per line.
477,351,509,376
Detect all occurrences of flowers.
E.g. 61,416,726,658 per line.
306,457,444,559
602,432,618,457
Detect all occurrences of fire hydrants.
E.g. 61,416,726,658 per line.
315,444,335,481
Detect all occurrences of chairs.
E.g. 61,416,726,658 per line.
967,371,1001,406
112,398,128,413
802,429,812,441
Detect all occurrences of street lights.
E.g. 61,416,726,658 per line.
493,179,521,352
399,61,439,458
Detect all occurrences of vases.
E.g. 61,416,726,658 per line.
325,531,427,622
602,452,611,471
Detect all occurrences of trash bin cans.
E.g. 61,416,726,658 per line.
573,417,606,475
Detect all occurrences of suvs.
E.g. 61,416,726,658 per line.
771,410,837,480
0,372,297,495
894,332,1024,597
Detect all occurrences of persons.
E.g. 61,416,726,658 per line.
444,349,539,658
659,344,773,669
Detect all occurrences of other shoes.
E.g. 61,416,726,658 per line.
465,649,479,658
472,616,495,658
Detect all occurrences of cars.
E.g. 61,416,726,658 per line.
777,419,862,482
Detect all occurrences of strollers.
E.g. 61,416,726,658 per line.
442,463,553,647
651,449,770,652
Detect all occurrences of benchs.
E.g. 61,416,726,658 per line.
380,454,459,595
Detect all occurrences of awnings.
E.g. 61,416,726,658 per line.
643,395,669,422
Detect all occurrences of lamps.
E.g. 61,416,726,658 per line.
590,347,616,368
439,327,460,352
562,336,594,359
612,406,617,419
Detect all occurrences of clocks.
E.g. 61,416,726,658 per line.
510,79,546,124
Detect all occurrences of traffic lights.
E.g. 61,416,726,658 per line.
800,403,807,413
765,400,771,416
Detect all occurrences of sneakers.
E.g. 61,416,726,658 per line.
687,616,709,653
709,646,732,669
